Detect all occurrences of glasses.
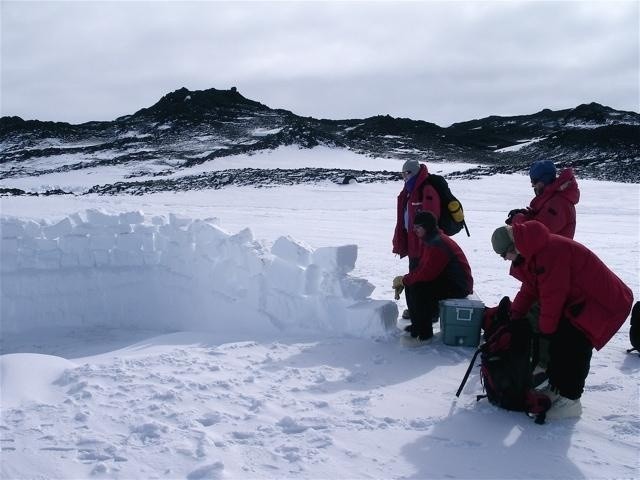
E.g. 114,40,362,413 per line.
500,248,507,258
402,171,410,179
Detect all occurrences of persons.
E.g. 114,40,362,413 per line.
505,159,580,239
491,220,634,418
393,159,440,322
392,211,473,348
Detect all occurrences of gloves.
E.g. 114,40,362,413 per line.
393,285,404,300
391,275,404,288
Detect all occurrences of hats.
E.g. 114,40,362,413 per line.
401,157,421,181
413,210,438,233
529,160,556,189
491,226,515,255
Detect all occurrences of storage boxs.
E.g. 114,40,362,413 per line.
440,288,489,347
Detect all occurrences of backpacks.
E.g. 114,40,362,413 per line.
626,301,640,356
456,317,551,425
417,174,470,237
481,296,513,335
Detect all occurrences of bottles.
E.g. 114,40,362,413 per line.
447,201,464,223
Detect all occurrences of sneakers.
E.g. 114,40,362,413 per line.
546,397,582,420
538,383,560,402
399,335,432,347
